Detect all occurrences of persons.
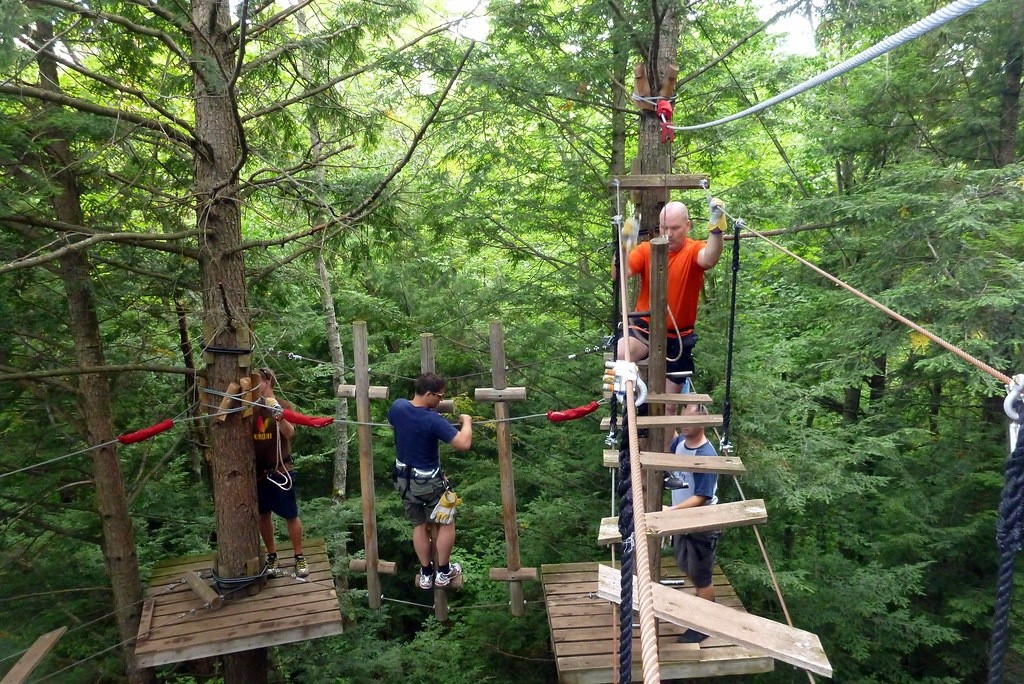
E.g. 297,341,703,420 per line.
612,198,728,489
253,368,309,577
388,373,473,590
668,402,719,643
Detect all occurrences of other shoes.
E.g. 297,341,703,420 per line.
682,627,708,644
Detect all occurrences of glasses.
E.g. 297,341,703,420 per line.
429,391,445,399
259,369,270,381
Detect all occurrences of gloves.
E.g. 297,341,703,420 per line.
621,217,640,251
447,493,462,524
429,491,456,524
706,198,728,233
272,404,284,422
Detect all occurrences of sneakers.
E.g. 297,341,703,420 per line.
267,553,279,570
435,563,461,586
420,562,433,589
662,471,689,491
295,557,310,577
